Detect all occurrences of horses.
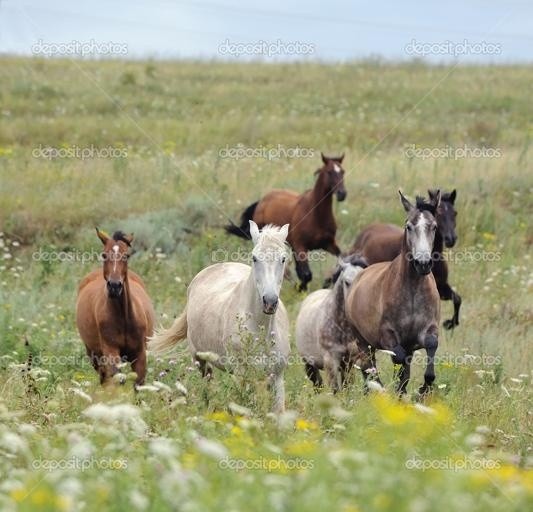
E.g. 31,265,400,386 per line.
222,152,348,292
344,188,441,403
75,226,156,392
294,249,373,397
349,187,462,330
144,219,290,417
